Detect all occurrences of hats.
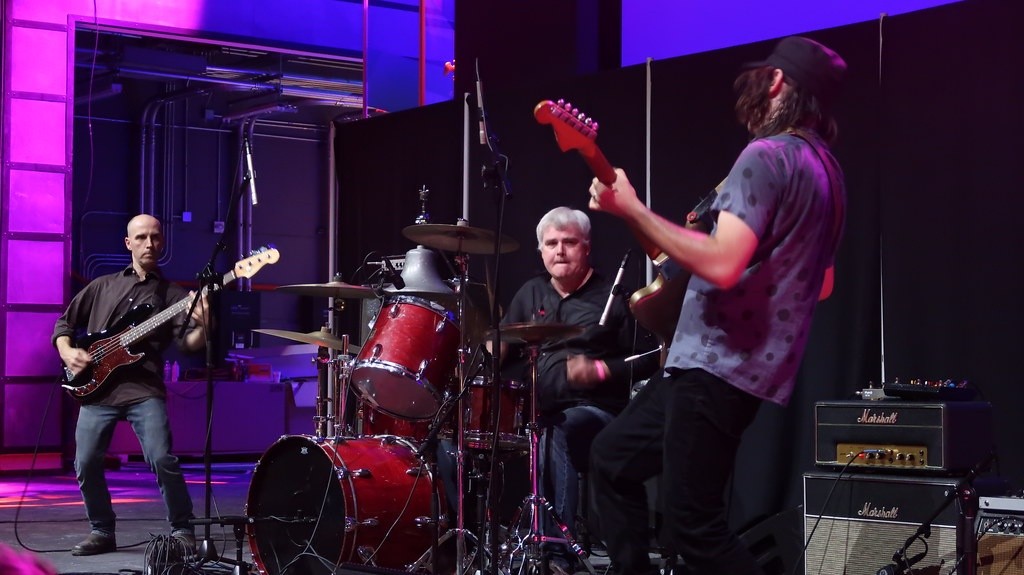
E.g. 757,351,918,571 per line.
749,35,847,101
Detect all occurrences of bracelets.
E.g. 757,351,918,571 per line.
594,358,606,383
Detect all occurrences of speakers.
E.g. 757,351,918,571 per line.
802,468,1024,575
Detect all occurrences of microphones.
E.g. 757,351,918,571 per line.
876,552,924,575
244,142,258,205
380,254,406,291
475,81,485,144
480,345,492,367
289,518,317,526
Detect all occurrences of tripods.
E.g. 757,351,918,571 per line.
406,106,599,575
179,171,251,573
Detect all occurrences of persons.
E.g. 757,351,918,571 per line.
587,36,849,575
50,214,211,556
437,206,635,553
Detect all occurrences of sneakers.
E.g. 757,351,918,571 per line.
173,529,195,556
71,531,116,556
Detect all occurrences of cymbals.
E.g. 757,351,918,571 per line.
485,322,583,343
402,223,520,256
276,279,378,299
250,327,361,354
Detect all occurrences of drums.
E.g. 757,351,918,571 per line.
446,375,538,451
309,355,432,453
347,295,470,422
245,433,443,575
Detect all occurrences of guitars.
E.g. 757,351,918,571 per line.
530,95,707,370
63,242,282,402
